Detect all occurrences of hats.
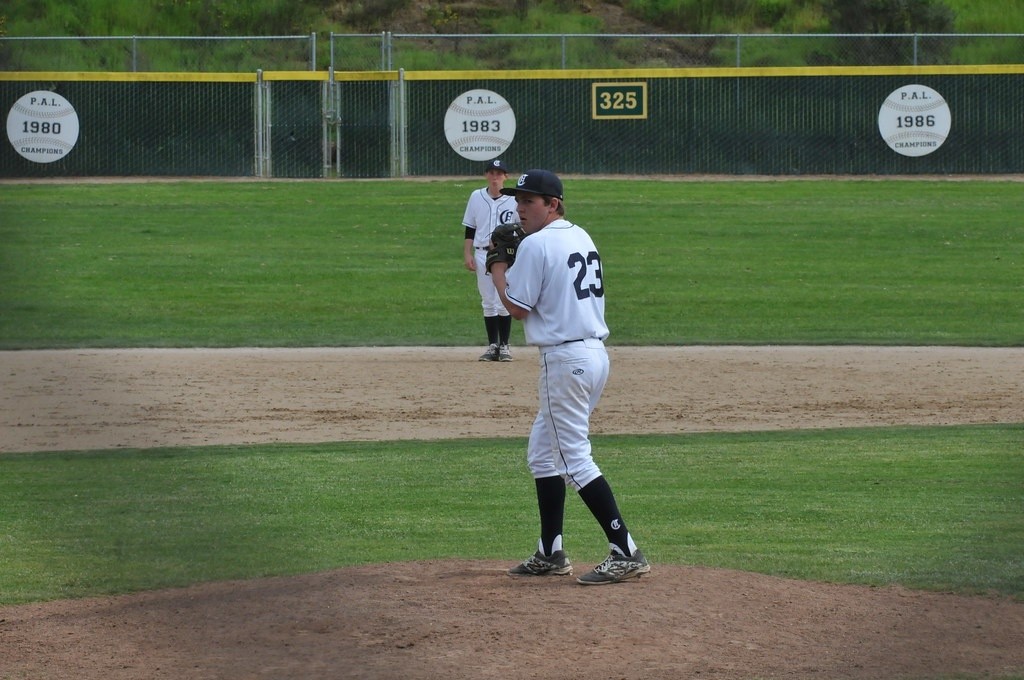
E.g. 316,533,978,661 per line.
486,160,506,172
498,169,564,203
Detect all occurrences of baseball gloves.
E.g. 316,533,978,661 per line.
485,222,527,271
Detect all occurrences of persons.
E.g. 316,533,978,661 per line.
485,168,650,587
461,160,522,361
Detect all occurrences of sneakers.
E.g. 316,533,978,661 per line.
479,343,500,361
498,341,513,362
507,549,573,576
577,548,651,584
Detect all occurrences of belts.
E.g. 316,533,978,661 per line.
564,337,602,344
476,246,489,251
